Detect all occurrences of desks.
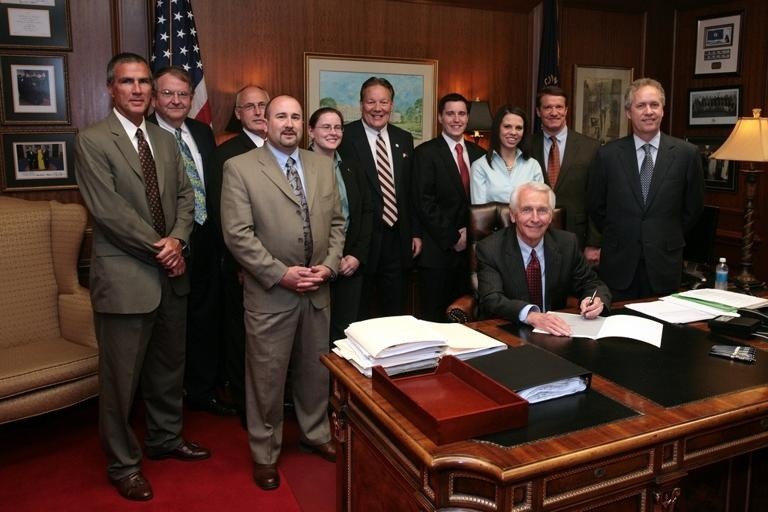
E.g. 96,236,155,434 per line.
319,287,768,512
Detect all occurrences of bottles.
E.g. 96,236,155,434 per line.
713,256,730,291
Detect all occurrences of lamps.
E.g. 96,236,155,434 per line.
708,108,768,296
464,96,495,146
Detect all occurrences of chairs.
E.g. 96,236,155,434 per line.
0,196,101,425
444,201,578,323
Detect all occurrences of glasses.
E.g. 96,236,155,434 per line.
160,88,190,101
316,123,344,133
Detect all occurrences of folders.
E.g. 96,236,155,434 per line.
461,344,592,406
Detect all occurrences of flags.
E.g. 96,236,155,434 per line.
150,1,214,131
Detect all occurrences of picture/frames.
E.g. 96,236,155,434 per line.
0,0,80,194
570,63,637,148
683,12,746,195
303,51,439,152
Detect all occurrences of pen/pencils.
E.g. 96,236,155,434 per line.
580,287,599,321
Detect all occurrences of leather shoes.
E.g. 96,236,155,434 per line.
301,439,337,462
114,473,151,500
153,440,211,462
195,393,240,417
255,460,279,491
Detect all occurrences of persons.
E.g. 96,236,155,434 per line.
693,94,736,116
412,93,488,324
74,52,376,501
18,70,50,105
521,86,602,250
334,76,414,321
475,184,612,337
585,80,706,304
470,104,544,205
25,146,50,170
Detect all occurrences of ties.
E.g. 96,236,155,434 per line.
526,250,544,310
376,132,400,228
547,136,561,188
454,144,470,197
135,129,168,239
285,159,314,266
640,142,656,204
174,126,209,228
